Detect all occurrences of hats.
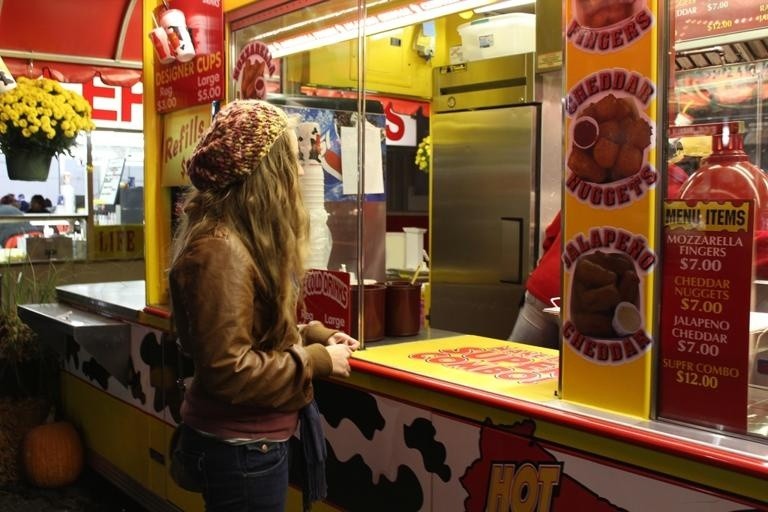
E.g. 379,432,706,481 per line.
184,98,291,193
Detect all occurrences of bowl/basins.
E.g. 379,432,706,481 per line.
571,0,646,31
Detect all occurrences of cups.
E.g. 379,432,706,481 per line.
347,278,422,344
570,116,600,150
294,121,333,271
611,302,642,337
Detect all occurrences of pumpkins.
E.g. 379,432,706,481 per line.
23,410,82,488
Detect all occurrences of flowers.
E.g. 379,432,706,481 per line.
415,135,430,173
0,73,93,160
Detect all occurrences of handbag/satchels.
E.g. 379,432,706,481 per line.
162,424,209,493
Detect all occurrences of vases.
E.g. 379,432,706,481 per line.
1,147,55,182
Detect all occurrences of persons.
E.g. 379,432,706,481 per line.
505,162,689,352
168,98,360,511
0,194,52,244
